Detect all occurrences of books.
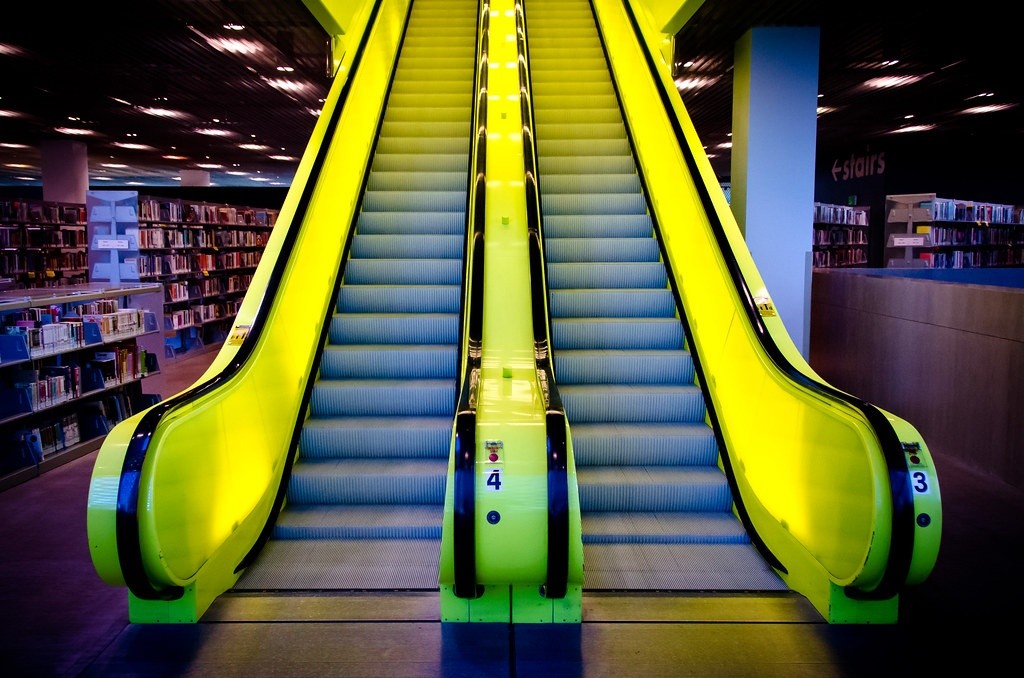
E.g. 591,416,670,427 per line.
0,200,279,477
812,200,1024,269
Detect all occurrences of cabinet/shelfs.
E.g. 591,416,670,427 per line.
1,194,280,365
812,193,1024,269
1,284,165,491
